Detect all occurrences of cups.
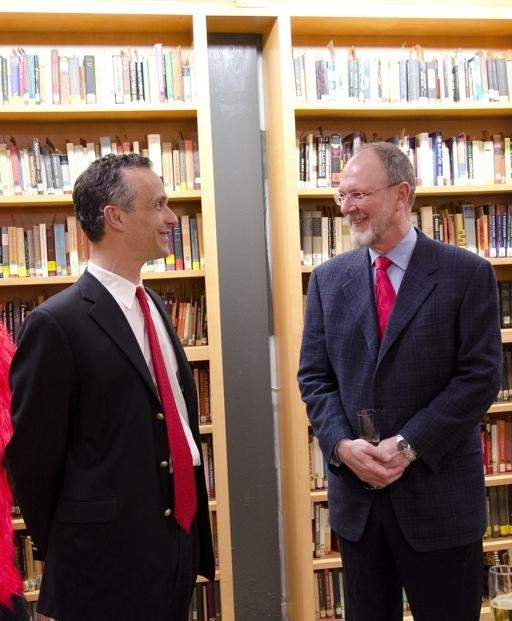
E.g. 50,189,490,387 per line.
489,565,512,621
356,409,380,489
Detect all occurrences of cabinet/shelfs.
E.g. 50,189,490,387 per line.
260,0,512,621
0,1,236,621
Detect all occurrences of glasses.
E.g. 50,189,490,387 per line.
336,180,401,206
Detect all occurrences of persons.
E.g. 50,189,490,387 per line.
0,321,30,621
1,154,215,621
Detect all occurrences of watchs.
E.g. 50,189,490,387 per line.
395,434,417,462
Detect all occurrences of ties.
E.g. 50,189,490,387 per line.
373,256,395,341
136,285,197,532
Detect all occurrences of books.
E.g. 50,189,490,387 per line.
297,142,504,621
0,295,55,621
189,435,222,621
412,202,512,258
403,586,411,612
152,284,212,426
497,280,512,329
479,350,512,602
296,129,512,189
293,54,512,103
0,212,93,279
1,133,201,196
309,426,346,619
4,44,192,107
140,212,206,273
298,202,360,266
